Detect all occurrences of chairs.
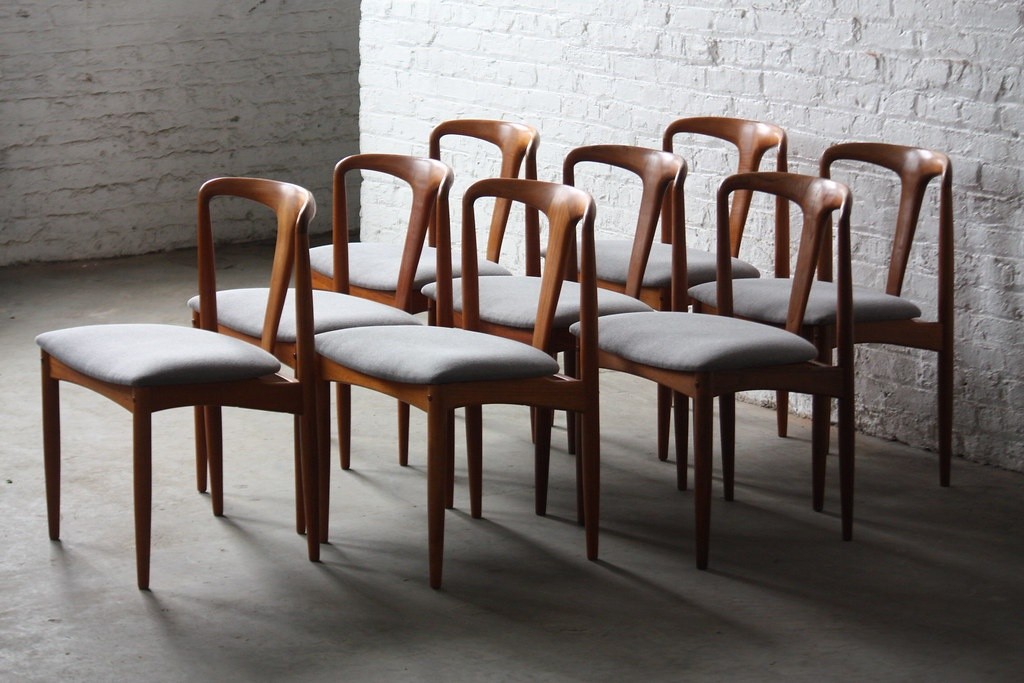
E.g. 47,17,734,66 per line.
541,116,791,463
567,171,853,571
420,145,689,530
34,176,321,590
308,119,543,465
686,141,955,515
298,176,602,591
187,154,455,535
